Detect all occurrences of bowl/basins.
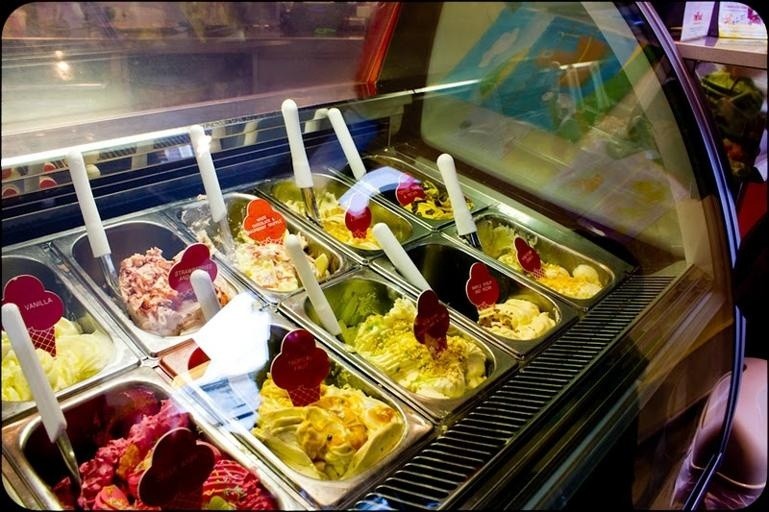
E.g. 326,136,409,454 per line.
272,271,522,423
333,150,485,227
254,170,424,261
165,192,352,302
2,243,129,416
53,215,262,351
3,368,309,512
151,309,431,506
436,210,630,313
366,235,581,356
